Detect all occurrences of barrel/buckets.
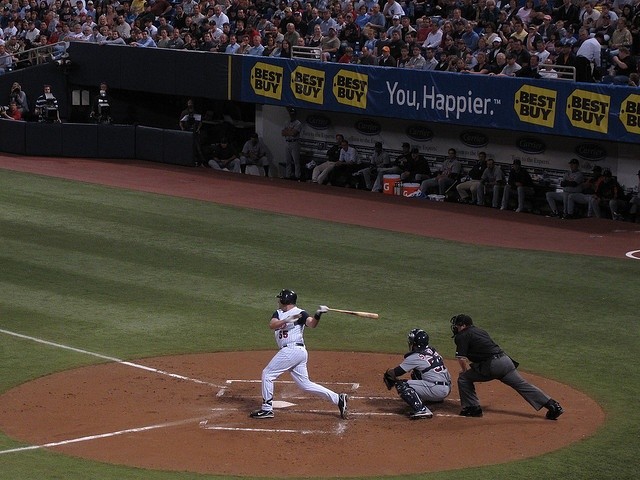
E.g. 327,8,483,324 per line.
403,183,420,196
384,175,399,193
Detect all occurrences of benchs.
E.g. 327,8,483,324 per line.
299,140,616,216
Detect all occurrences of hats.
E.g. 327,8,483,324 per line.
401,142,410,149
133,26,142,35
591,165,602,171
293,11,302,18
568,158,579,166
410,148,419,155
513,159,521,167
542,15,551,21
381,46,390,53
392,14,401,20
493,36,502,43
87,0,93,5
284,7,292,13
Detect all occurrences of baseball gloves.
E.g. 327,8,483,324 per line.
383,369,397,390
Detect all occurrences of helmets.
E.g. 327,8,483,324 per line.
406,329,429,352
450,314,473,338
276,288,297,305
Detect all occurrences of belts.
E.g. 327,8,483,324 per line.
492,354,504,360
433,381,449,386
280,343,304,349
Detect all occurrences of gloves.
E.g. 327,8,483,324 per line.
316,305,329,316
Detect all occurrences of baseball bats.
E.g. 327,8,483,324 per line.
328,308,380,319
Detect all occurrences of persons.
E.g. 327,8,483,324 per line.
456,152,488,204
90,82,113,122
35,85,62,124
449,314,564,420
0,0,204,75
384,328,451,420
353,142,391,189
2,101,22,120
545,159,583,220
499,159,536,212
477,159,508,208
629,169,640,223
250,289,346,419
609,178,626,220
312,134,343,184
280,108,303,179
331,140,362,186
568,166,603,218
240,133,269,177
209,138,242,173
10,82,28,113
400,149,431,182
419,149,461,194
204,0,418,70
371,142,410,192
178,98,208,168
418,0,640,87
587,170,617,217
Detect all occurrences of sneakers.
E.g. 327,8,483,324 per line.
410,405,433,420
545,209,559,218
338,393,348,420
459,405,483,417
546,405,563,420
250,410,275,419
